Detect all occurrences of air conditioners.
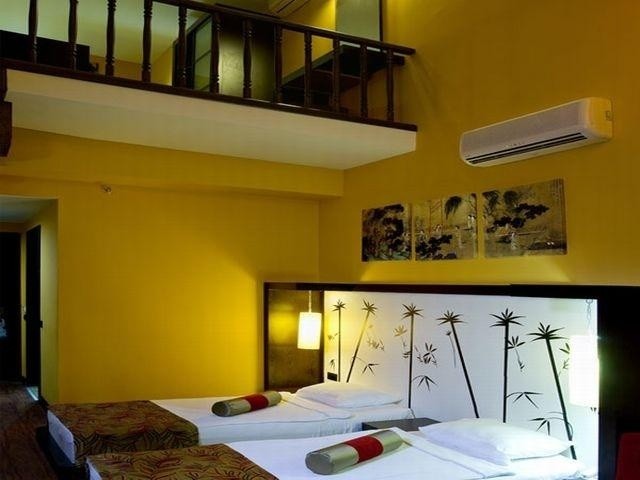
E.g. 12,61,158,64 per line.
456,95,613,168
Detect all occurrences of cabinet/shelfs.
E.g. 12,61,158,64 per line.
172,3,277,99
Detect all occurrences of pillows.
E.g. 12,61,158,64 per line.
211,379,404,418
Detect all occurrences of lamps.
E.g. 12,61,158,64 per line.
297,289,323,351
